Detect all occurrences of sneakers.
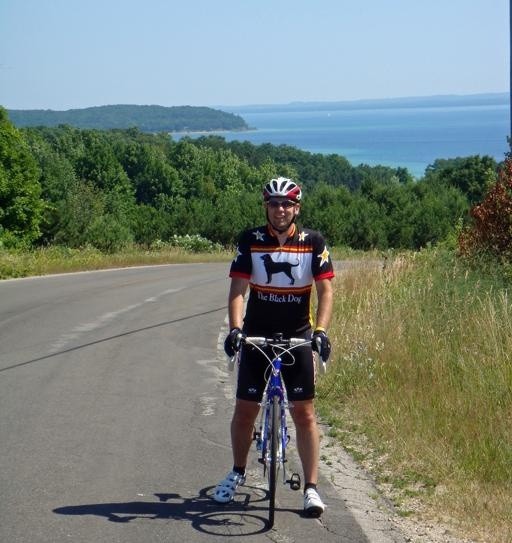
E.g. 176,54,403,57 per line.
214,471,247,503
303,488,324,517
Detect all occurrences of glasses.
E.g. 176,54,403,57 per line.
268,200,296,208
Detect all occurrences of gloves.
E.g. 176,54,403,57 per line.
312,330,331,362
224,328,242,357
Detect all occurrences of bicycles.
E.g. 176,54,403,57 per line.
227,329,327,529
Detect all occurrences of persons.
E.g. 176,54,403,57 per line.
211,177,336,516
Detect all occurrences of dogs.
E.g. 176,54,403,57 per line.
260,253,300,286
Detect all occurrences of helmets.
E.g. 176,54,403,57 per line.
264,177,302,202
